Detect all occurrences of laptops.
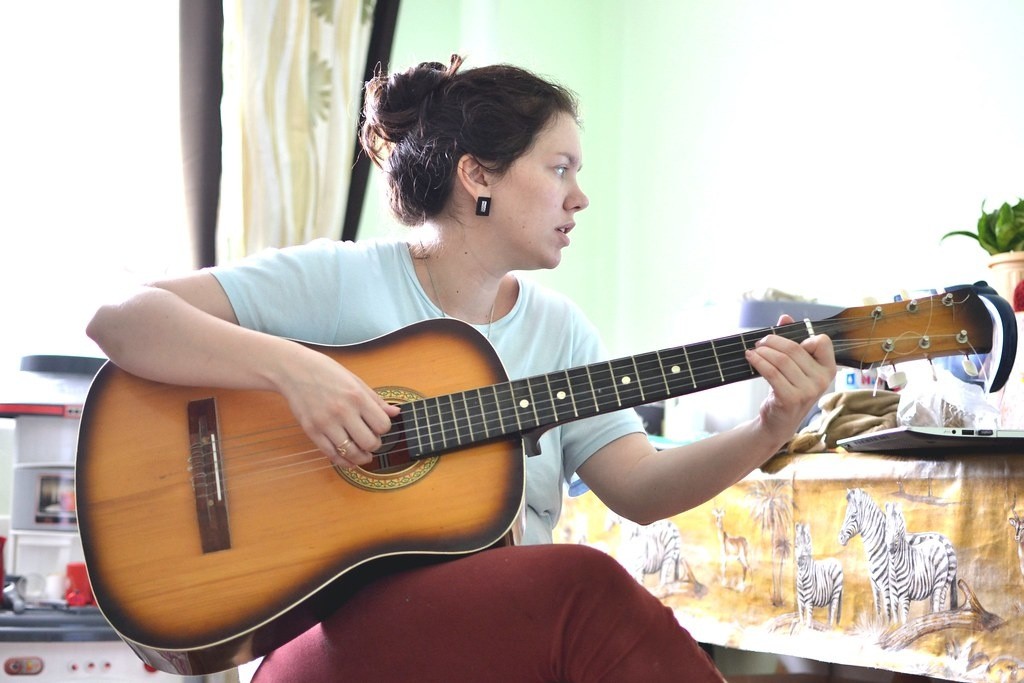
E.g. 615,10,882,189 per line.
835,424,1024,453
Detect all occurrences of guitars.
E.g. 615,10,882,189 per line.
74,285,993,676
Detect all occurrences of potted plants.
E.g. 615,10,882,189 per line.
940,196,1024,311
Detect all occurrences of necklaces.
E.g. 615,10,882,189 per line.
418,240,495,340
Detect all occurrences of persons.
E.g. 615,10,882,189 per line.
85,51,838,683
